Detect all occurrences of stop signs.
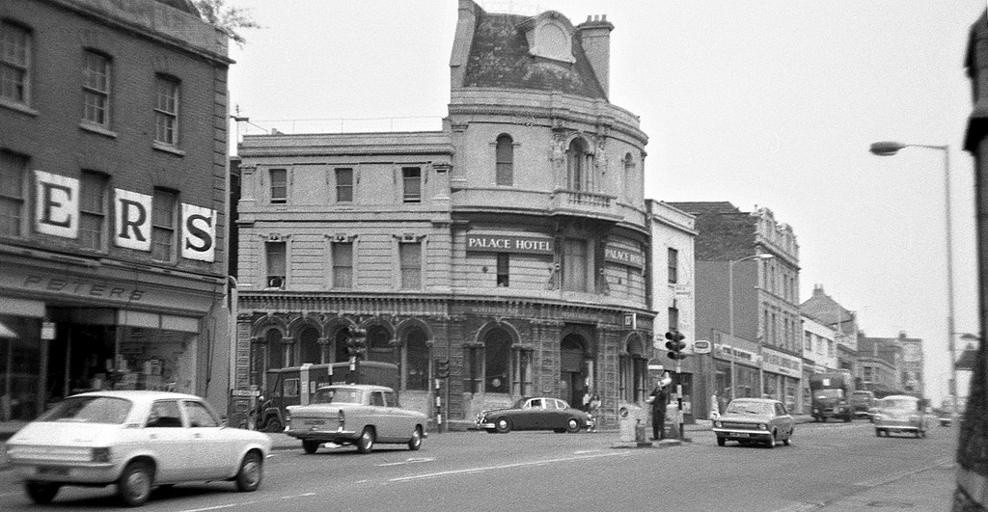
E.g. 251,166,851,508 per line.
646,357,665,378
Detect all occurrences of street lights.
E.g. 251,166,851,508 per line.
728,252,775,398
864,135,962,429
961,331,981,343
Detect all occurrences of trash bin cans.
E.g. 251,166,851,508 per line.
227,387,255,428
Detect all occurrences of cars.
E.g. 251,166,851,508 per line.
3,388,276,510
278,383,433,458
711,395,797,449
472,394,597,435
937,404,969,426
873,394,929,439
852,388,875,421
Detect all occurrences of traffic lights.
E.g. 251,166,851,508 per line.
664,330,678,361
676,330,686,361
434,357,451,380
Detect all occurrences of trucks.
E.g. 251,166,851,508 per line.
807,366,856,423
255,355,405,434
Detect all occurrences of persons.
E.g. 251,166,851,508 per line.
582,389,603,433
644,372,672,441
710,390,729,420
